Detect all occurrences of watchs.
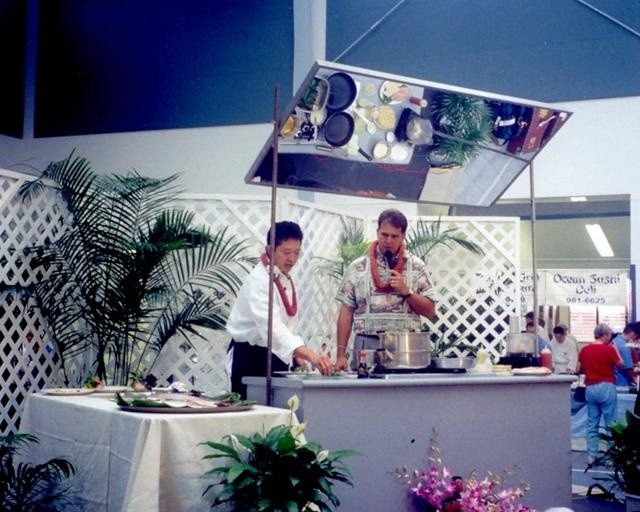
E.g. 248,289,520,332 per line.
402,288,413,299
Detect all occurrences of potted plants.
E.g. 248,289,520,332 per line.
583,392,640,512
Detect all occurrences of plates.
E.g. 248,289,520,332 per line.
378,79,410,105
385,131,395,143
512,366,553,376
116,394,253,413
373,105,396,130
41,387,133,395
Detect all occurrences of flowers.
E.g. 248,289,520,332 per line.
389,425,534,512
197,395,364,512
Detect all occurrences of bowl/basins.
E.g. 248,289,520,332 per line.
391,142,409,162
307,106,327,126
373,140,391,161
494,364,514,378
407,117,434,147
280,114,300,139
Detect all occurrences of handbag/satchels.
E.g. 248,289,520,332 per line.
574,386,585,401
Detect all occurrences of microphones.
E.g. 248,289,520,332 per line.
384,250,394,272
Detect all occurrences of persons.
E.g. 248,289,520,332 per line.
525,311,640,388
332,208,439,372
576,323,624,465
225,221,333,401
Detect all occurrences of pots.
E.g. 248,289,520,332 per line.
322,112,354,148
374,327,432,371
324,71,357,112
432,354,479,368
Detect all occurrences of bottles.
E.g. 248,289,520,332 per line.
358,351,369,379
410,95,427,108
541,344,553,374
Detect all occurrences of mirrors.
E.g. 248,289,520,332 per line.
244,58,575,208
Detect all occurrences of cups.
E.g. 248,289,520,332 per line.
501,333,538,368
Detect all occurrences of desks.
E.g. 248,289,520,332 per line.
27,385,293,512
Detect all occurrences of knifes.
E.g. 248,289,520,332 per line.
315,145,353,159
